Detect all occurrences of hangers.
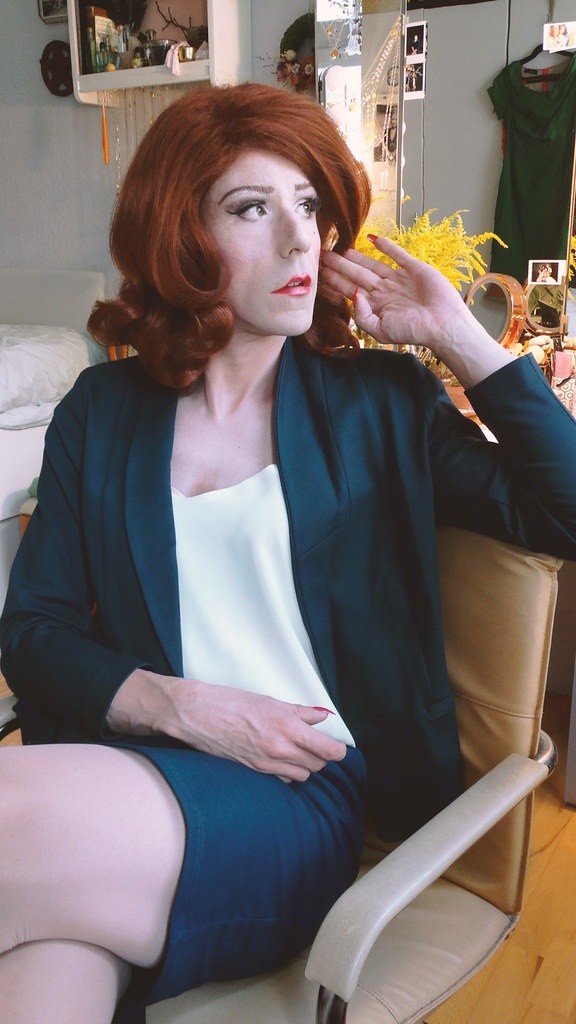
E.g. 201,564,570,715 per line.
520,12,573,84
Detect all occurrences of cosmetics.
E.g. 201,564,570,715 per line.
82,24,198,74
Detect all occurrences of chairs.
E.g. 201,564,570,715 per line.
0,523,565,1023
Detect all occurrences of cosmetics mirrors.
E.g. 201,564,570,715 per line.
521,276,572,336
464,274,527,350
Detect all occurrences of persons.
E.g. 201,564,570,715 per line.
536,264,557,283
0,85,576,1024
545,24,570,48
410,35,421,54
384,113,397,152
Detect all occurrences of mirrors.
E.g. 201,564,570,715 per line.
395,0,576,341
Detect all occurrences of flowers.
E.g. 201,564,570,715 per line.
252,49,315,92
